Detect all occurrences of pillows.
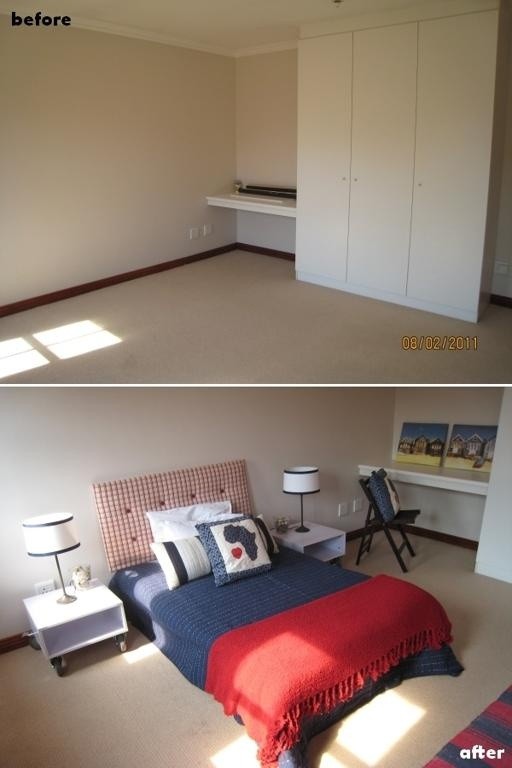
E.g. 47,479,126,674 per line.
363,466,401,522
137,500,279,590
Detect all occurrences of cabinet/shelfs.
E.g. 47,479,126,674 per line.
293,1,511,326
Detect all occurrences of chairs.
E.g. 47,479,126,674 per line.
355,472,423,574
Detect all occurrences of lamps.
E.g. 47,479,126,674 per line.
20,508,83,606
282,465,319,533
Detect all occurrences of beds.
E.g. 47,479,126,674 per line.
91,459,462,768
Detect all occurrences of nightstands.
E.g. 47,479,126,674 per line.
270,523,347,571
20,583,130,679
